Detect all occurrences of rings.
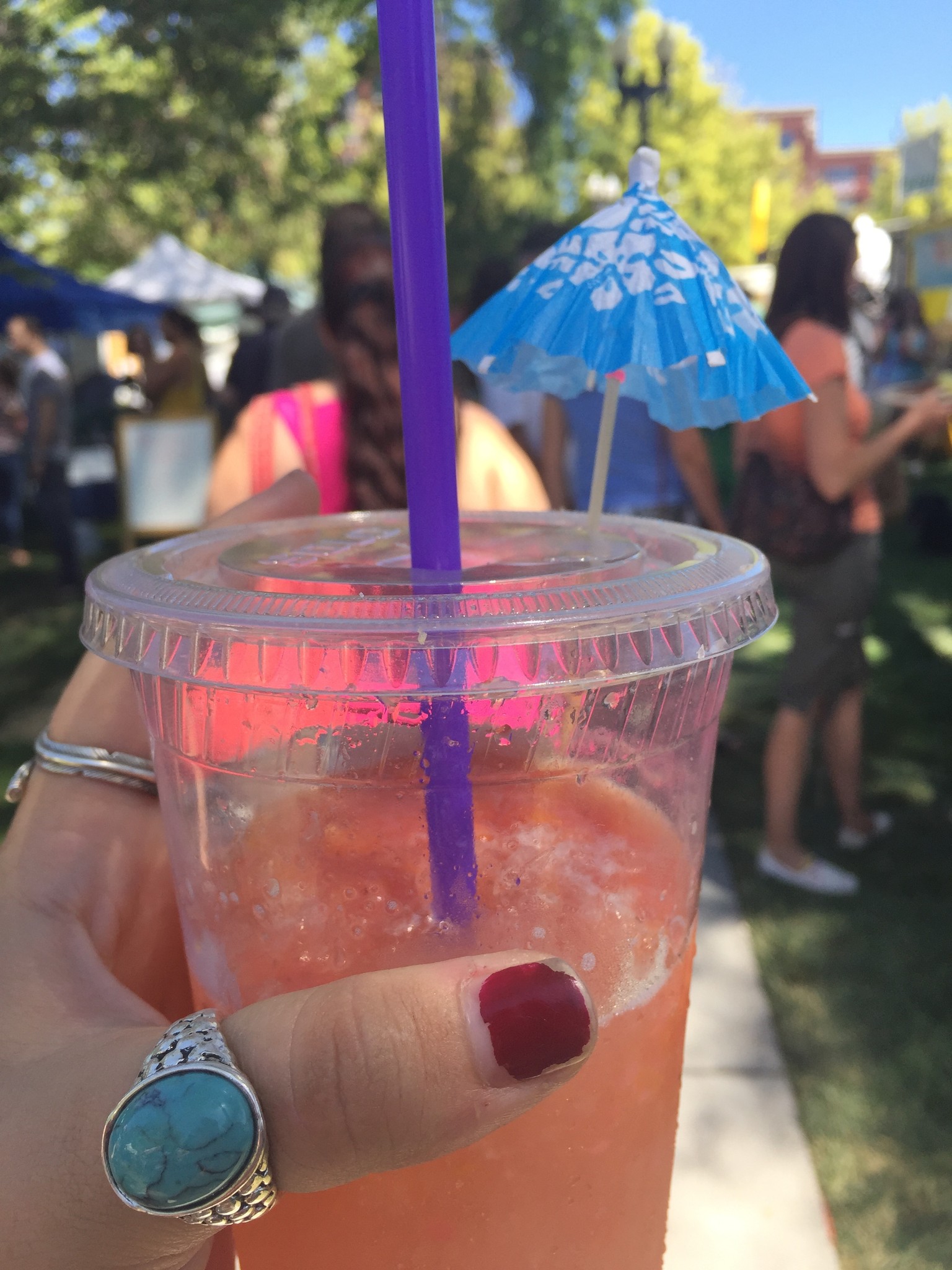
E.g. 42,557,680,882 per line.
101,1008,278,1227
5,724,159,806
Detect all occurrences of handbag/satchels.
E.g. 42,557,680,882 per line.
734,448,853,563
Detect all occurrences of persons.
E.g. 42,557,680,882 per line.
0,202,952,899
0,471,598,1270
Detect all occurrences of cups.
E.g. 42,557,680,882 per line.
76,505,780,1268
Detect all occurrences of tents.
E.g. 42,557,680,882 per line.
102,233,266,317
0,237,174,345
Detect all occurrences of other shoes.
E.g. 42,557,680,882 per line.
758,840,858,899
837,812,891,850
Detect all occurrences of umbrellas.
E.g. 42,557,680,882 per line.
450,146,819,531
